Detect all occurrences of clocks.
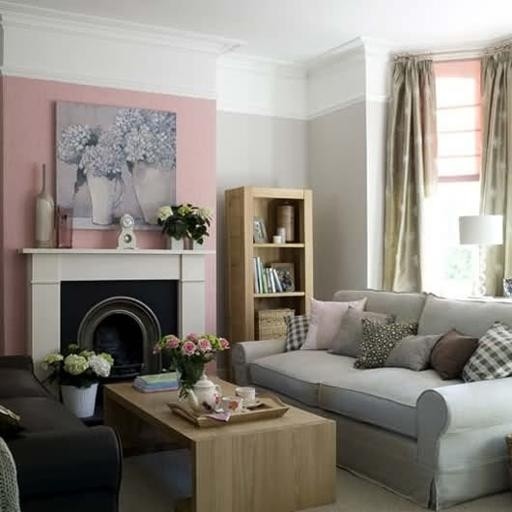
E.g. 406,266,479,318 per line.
116,214,139,251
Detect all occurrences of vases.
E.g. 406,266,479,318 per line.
32,163,55,249
56,204,74,249
85,169,128,225
130,160,174,227
176,379,206,402
164,228,184,250
60,383,98,419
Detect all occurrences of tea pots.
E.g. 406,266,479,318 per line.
184,374,223,414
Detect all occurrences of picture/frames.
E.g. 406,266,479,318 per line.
272,261,295,291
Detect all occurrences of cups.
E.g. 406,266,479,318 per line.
221,396,246,415
271,236,282,245
235,385,256,405
275,227,286,244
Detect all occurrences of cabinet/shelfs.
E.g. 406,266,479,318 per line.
225,186,314,344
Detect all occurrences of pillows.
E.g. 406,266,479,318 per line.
461,320,512,384
327,304,399,357
383,332,444,372
300,294,368,351
354,317,419,370
283,314,310,353
428,325,480,380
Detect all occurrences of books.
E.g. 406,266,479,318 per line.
133,371,182,393
254,217,287,293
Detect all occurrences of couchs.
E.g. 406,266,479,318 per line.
0,352,124,512
231,289,512,512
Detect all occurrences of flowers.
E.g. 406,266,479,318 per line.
156,201,215,246
151,331,231,397
114,107,176,177
38,344,114,406
58,124,127,200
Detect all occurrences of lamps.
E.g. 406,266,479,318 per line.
458,215,505,299
